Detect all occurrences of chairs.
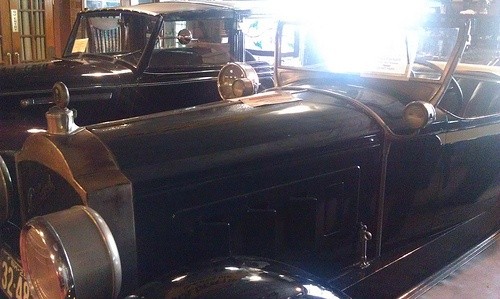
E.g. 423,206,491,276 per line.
147,50,202,67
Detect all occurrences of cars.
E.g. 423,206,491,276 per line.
1,2,500,299
